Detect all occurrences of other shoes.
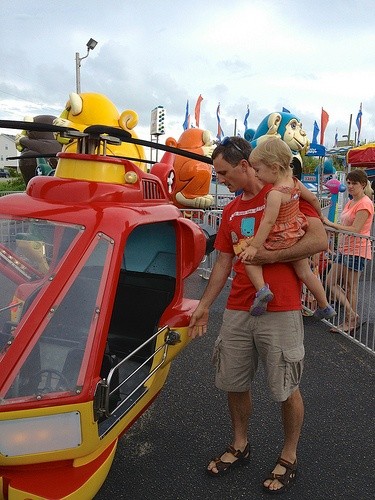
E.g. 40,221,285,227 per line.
302,310,313,317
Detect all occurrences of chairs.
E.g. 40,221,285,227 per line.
65,262,178,359
14,279,115,400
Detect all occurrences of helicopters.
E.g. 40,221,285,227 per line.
0,118,216,499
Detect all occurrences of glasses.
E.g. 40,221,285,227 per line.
220,137,247,156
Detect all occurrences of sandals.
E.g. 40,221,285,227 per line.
208,442,251,476
263,457,298,494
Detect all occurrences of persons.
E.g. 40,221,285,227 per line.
188,136,329,491
238,137,337,320
323,169,374,331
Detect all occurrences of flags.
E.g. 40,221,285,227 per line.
283,108,290,113
356,103,362,134
312,121,319,144
216,105,224,140
244,108,249,130
183,103,190,130
195,96,203,127
335,133,338,146
320,109,329,144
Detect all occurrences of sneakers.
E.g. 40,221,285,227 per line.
250,287,273,316
313,305,336,322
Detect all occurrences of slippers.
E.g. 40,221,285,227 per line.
330,323,349,333
347,320,367,334
